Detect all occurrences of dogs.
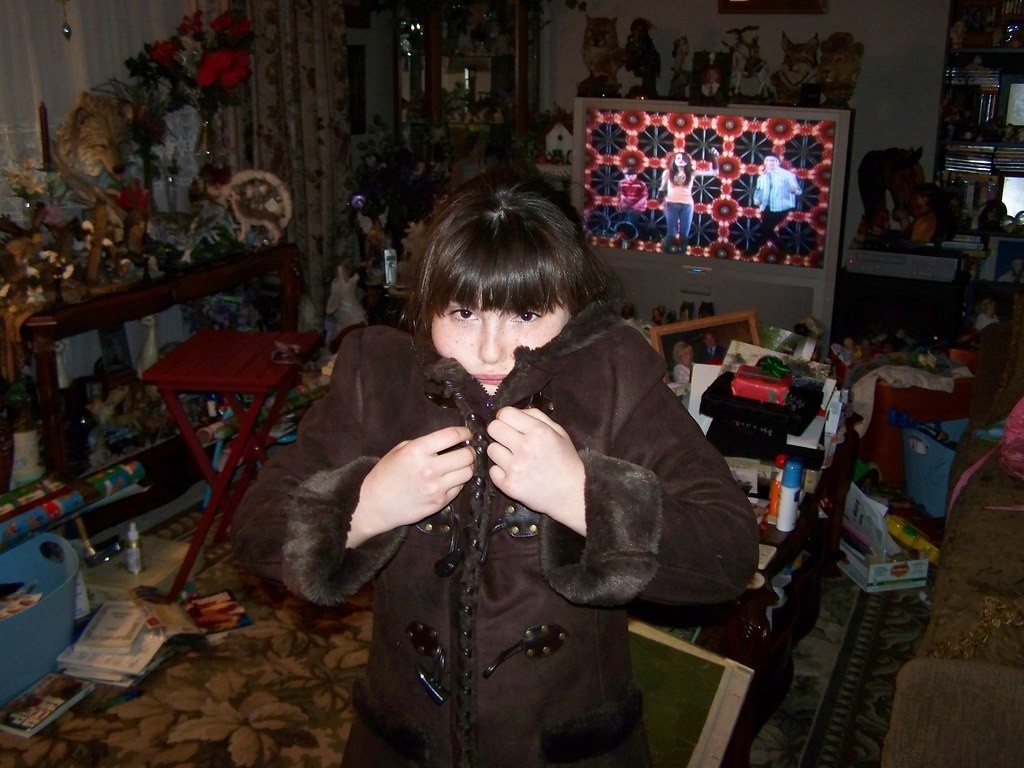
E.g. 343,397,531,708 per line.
326,265,368,339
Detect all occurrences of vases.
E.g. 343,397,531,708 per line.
192,121,226,172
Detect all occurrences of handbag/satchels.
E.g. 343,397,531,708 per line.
1001,397,1024,482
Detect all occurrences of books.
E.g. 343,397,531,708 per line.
937,0,1024,229
0,581,255,739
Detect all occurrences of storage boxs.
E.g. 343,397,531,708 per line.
836,538,928,592
837,348,977,472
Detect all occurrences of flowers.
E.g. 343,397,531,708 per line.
125,9,259,121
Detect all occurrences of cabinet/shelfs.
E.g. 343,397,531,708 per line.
392,0,528,167
14,242,302,541
846,271,972,347
933,0,1024,239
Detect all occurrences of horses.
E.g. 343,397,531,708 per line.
858,145,926,218
728,51,774,98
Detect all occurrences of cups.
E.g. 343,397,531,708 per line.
13,430,47,483
688,51,733,107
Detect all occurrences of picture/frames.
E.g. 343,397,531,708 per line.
649,311,761,384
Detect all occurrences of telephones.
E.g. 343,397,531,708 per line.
382,248,406,293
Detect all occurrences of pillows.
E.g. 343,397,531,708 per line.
980,294,1024,429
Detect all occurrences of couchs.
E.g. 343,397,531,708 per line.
880,323,1024,767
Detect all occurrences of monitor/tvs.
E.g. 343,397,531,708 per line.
572,98,853,281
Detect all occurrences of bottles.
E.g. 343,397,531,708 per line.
126,522,142,574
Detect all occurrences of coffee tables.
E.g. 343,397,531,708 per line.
630,411,863,768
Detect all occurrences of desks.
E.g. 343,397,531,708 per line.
143,328,323,605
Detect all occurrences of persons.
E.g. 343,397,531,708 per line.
972,256,1024,333
614,66,803,264
668,330,723,385
218,187,760,768
